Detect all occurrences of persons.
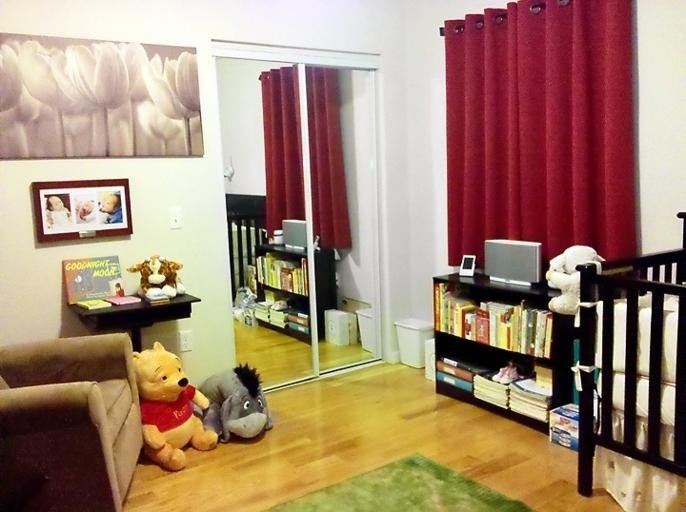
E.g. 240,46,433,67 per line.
46,196,72,228
78,193,121,223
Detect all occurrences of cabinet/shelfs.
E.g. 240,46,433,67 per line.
431,271,574,435
249,246,338,347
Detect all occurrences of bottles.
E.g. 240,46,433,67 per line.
272,229,283,246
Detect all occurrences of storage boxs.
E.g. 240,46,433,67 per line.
549,403,580,451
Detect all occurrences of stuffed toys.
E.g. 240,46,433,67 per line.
193,361,273,444
132,341,218,472
546,245,606,316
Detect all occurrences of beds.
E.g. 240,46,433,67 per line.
570,212,686,512
226,193,266,306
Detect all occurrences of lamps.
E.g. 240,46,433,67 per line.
224,156,234,178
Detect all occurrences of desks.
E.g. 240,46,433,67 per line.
70,296,201,353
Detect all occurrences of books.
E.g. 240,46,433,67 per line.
435,283,552,424
254,302,309,334
257,252,309,297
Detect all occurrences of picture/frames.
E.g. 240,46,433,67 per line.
32,178,133,243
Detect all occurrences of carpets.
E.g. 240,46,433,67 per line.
256,450,536,511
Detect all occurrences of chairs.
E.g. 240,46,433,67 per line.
0,332,145,512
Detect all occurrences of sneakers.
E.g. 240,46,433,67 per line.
491,366,518,384
